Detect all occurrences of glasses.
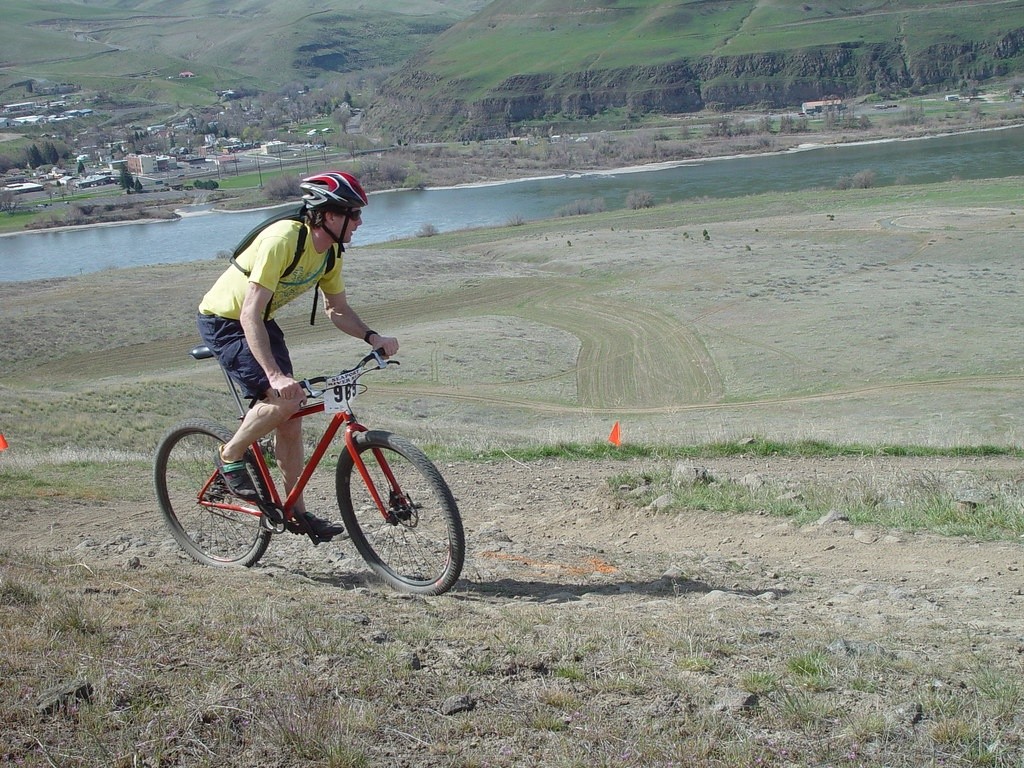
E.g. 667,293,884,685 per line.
349,210,362,221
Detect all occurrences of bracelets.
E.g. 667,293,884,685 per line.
365,331,377,344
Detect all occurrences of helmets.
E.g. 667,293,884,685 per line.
300,171,369,212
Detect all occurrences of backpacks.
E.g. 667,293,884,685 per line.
229,205,336,281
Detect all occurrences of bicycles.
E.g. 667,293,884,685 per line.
153,339,467,599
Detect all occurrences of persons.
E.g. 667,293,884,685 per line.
197,172,399,536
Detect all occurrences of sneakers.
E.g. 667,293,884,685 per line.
305,518,343,545
213,444,259,502
